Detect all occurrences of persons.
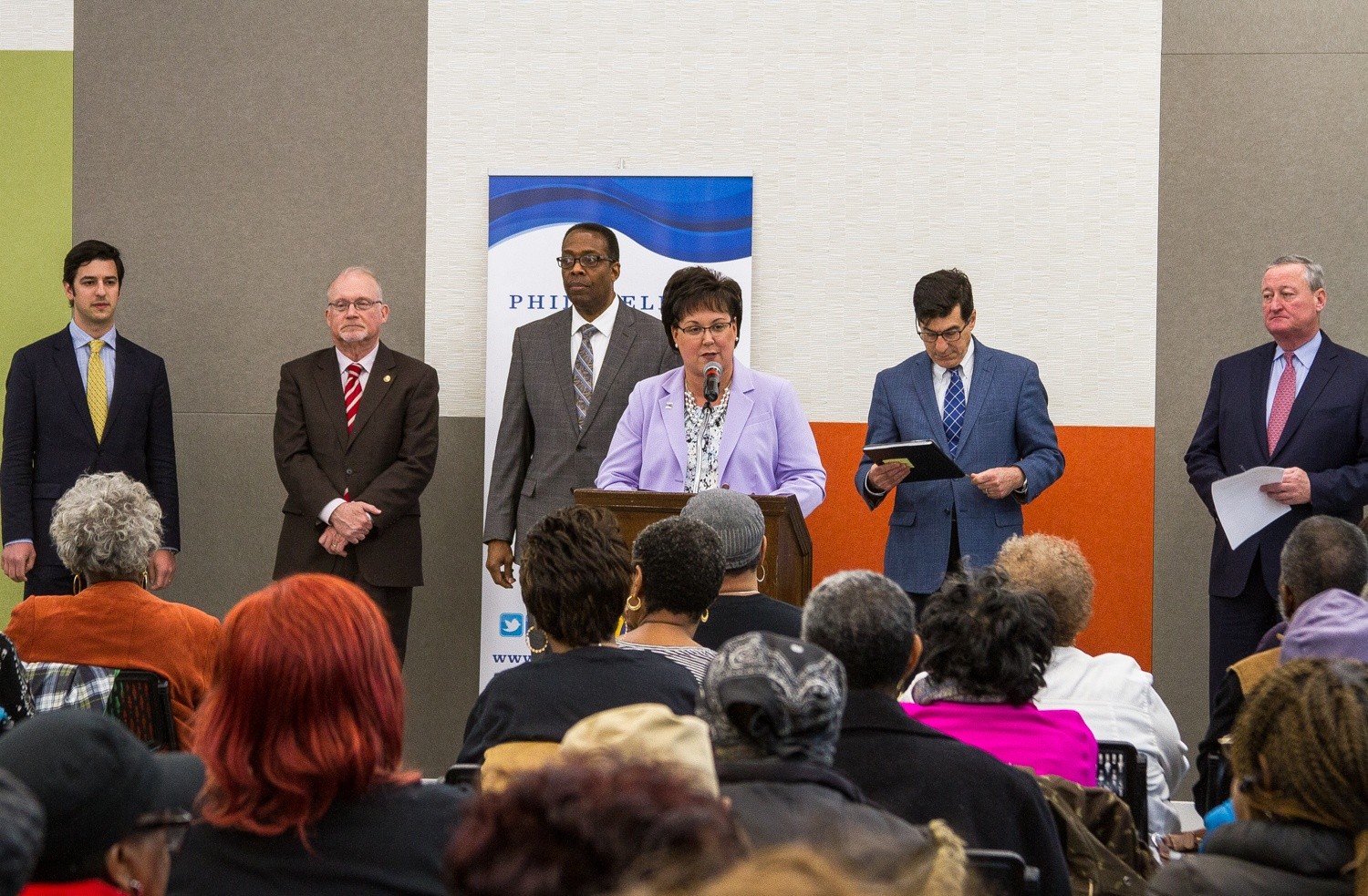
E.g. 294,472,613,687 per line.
853,267,1067,624
593,264,827,519
0,469,1368,896
1183,252,1368,820
272,265,440,675
484,222,684,658
0,239,182,602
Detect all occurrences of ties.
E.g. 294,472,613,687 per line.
572,323,600,436
341,362,364,436
1267,351,1297,459
85,340,109,446
943,365,966,460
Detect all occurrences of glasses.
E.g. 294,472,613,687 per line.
675,314,736,339
556,255,615,270
915,313,970,342
112,803,192,852
327,297,382,311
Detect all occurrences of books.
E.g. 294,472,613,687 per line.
862,439,964,483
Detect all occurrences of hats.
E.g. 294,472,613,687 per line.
681,488,766,570
0,708,204,878
562,704,722,800
704,632,846,773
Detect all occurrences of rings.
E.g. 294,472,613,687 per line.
985,488,988,495
331,543,336,548
168,579,171,582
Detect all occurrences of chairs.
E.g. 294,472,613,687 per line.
19,661,181,753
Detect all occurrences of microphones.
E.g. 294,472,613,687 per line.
703,361,723,401
